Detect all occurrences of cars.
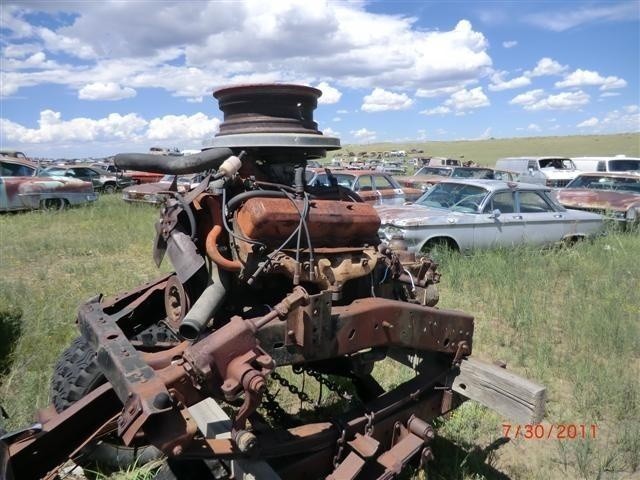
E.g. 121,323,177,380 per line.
305,150,513,207
0,148,207,214
373,177,610,261
538,172,640,234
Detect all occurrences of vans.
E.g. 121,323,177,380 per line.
494,155,584,187
570,155,639,184
